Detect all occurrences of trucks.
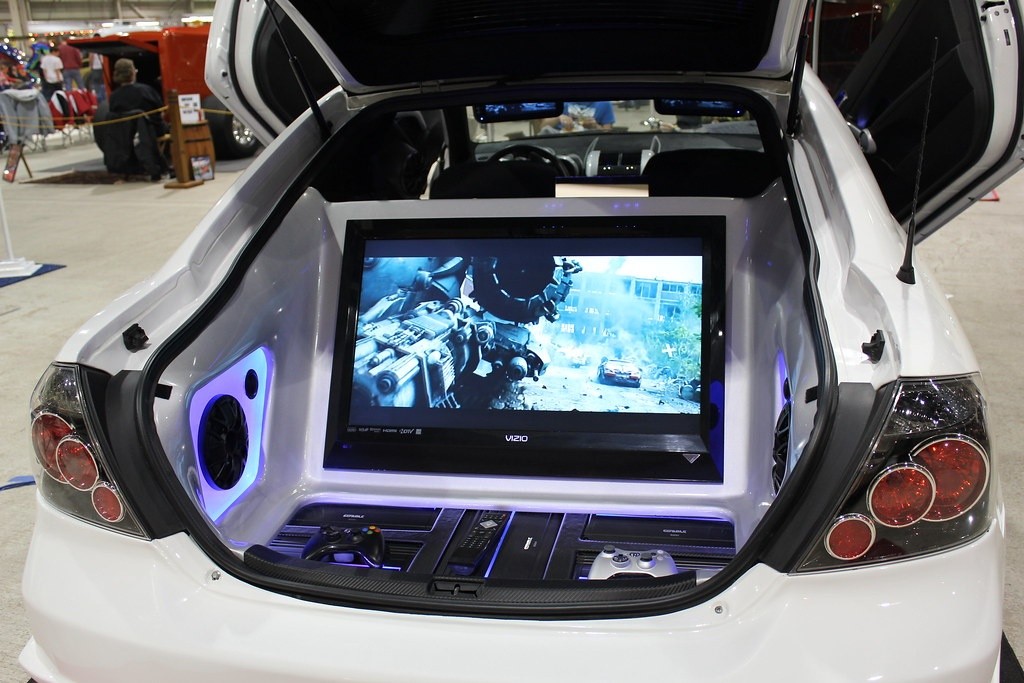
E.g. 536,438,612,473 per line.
65,17,260,162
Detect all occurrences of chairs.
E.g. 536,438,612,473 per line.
111,134,170,180
644,148,772,198
429,163,557,202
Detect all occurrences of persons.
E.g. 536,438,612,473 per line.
40,47,65,97
89,33,104,95
57,36,85,90
109,58,173,179
540,102,616,133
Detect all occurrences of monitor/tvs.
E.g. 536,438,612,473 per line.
322,216,726,486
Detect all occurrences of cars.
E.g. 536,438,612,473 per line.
17,0,1023,683
